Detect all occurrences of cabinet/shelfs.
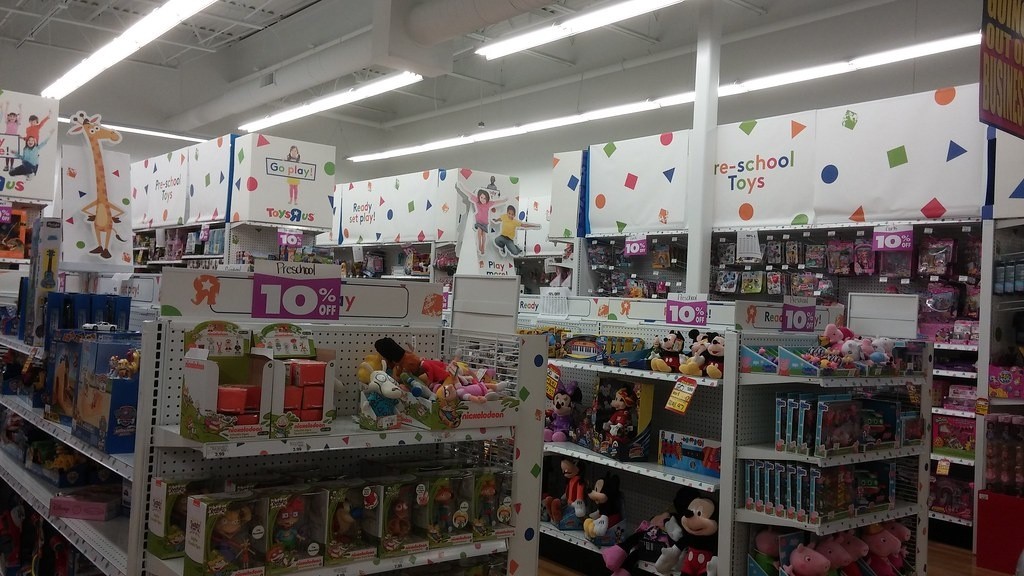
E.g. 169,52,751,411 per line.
318,242,457,282
444,292,935,576
131,218,330,273
0,197,50,264
0,267,551,576
582,217,1024,559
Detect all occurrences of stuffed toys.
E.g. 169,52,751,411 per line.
357,337,507,404
543,324,911,576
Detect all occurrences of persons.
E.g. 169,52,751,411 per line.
3,101,56,179
456,175,541,257
287,145,300,206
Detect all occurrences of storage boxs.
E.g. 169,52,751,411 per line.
0,207,1024,576
